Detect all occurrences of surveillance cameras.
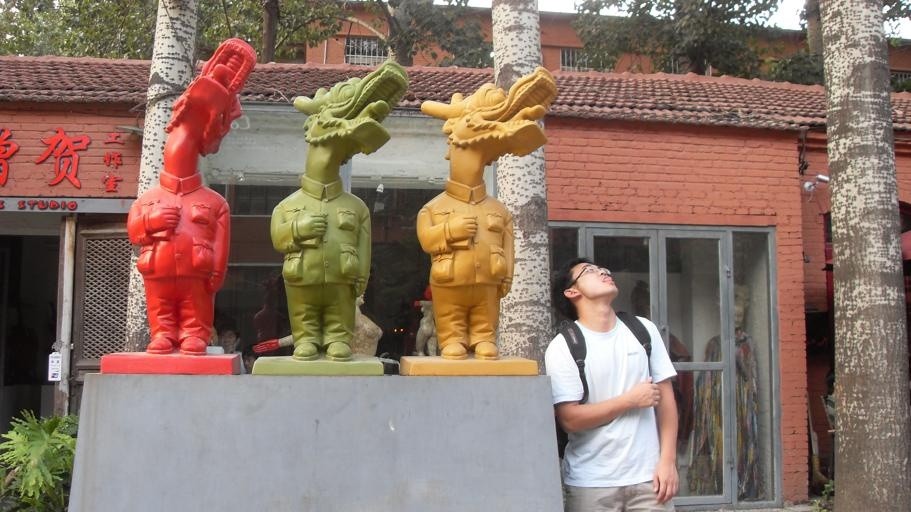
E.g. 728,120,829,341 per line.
817,174,830,183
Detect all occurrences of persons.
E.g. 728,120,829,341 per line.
686,287,759,502
544,257,682,512
214,323,247,373
241,344,264,374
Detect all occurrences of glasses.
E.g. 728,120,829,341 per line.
570,265,612,288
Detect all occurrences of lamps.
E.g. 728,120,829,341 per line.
803,175,830,192
